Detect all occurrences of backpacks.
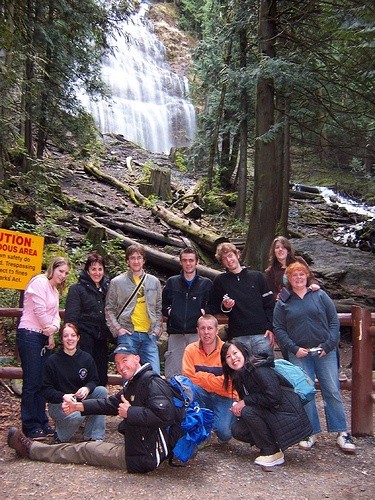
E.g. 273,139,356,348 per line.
141,371,214,463
251,359,316,406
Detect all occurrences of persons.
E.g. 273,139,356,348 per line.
220,341,313,466
17,257,72,440
42,323,107,441
213,242,275,363
273,262,356,451
8,344,177,470
265,237,320,297
182,314,240,442
105,245,162,386
65,254,107,387
162,249,213,377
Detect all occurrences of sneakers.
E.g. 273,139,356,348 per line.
299,434,316,451
254,450,284,466
336,433,356,451
7,427,32,458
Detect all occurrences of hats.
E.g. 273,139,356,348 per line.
109,344,138,360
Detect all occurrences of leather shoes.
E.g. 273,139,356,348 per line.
43,426,55,437
29,429,46,440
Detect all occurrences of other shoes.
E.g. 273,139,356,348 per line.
198,441,210,449
217,438,229,443
339,372,346,381
